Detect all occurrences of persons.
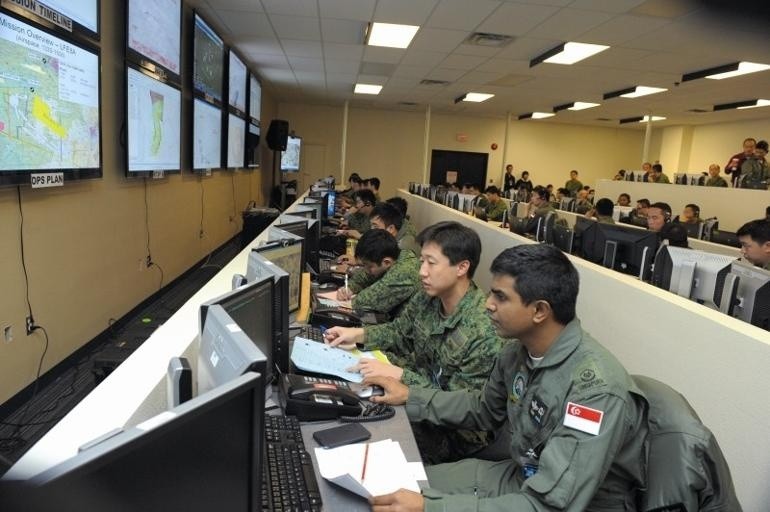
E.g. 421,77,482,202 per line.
587,193,703,264
504,164,533,204
706,164,729,187
437,183,507,222
524,185,559,226
724,137,770,190
736,218,770,330
642,162,669,184
364,244,651,512
323,220,514,465
546,170,594,217
336,173,422,258
336,228,424,319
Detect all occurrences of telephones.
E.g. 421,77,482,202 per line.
310,303,362,328
326,218,342,227
318,270,350,287
277,371,362,422
318,246,336,259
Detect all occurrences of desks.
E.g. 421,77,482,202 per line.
265,229,432,512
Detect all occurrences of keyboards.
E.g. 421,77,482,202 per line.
261,413,325,512
301,326,324,343
319,257,336,273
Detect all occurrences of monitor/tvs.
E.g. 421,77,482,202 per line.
124,59,183,178
0,0,101,53
225,49,262,168
250,240,304,315
269,189,336,277
407,181,572,252
190,92,225,172
574,214,660,284
730,261,770,328
245,252,290,353
200,275,278,386
191,9,224,107
191,306,268,399
14,371,264,511
652,245,741,311
0,6,105,188
124,0,183,87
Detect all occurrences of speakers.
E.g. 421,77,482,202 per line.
266,120,289,151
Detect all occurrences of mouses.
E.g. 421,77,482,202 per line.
355,383,385,398
319,282,339,290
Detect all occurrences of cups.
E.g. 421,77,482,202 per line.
346,239,358,257
732,158,740,171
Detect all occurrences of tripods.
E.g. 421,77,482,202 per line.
269,150,283,212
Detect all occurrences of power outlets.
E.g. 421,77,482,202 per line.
145,256,155,270
25,315,40,336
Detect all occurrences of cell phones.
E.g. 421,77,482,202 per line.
312,422,372,450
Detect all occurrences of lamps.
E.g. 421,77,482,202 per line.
529,41,611,69
680,61,768,82
552,101,600,112
620,116,667,125
713,99,769,112
518,111,555,120
602,86,670,100
453,92,495,105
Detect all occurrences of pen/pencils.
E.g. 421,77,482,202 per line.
320,325,345,345
345,273,349,302
361,445,368,480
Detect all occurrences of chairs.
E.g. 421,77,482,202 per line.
621,372,714,511
